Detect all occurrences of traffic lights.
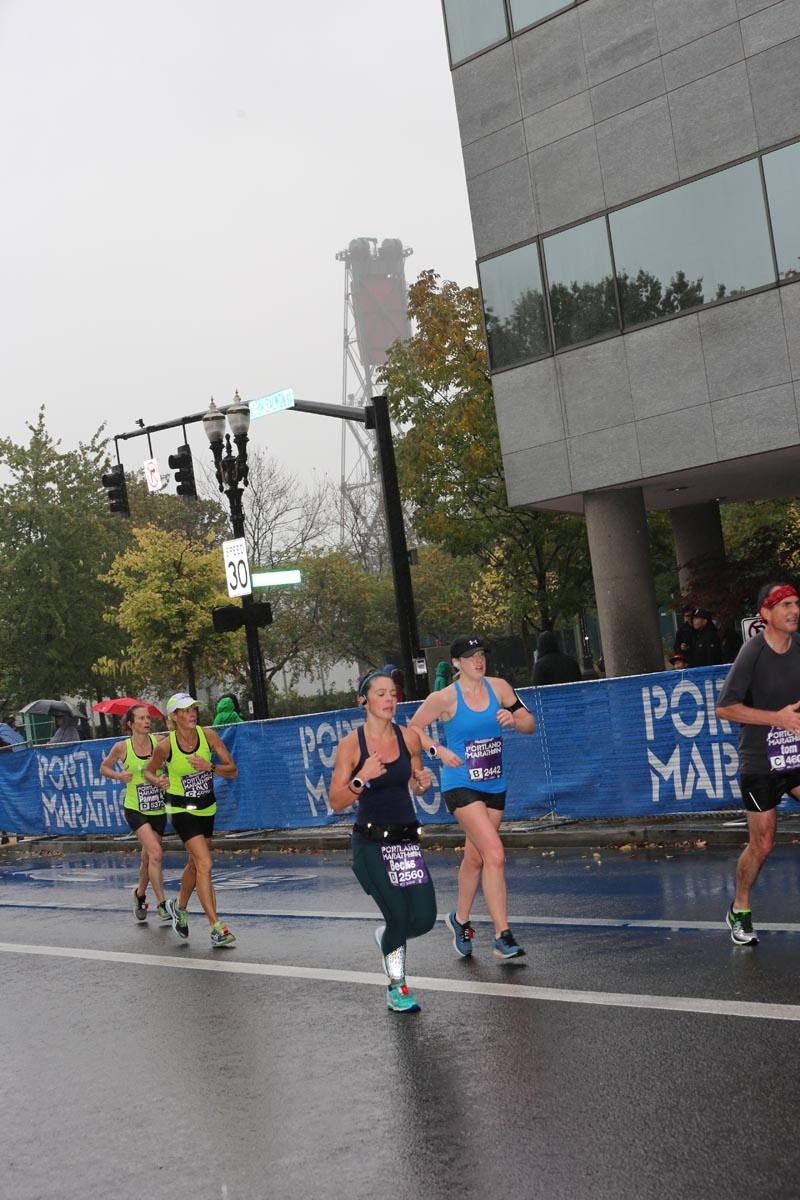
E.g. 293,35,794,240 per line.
168,443,198,501
102,463,132,519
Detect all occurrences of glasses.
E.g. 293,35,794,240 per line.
683,614,693,618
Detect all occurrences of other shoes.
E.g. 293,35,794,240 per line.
130,888,148,920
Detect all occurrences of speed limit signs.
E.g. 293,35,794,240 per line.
222,537,253,599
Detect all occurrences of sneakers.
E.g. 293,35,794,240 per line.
386,982,420,1014
726,903,761,945
209,920,239,948
157,900,174,920
165,897,190,939
493,929,527,959
374,926,401,978
445,912,475,957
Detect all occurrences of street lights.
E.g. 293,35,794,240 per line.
202,387,274,721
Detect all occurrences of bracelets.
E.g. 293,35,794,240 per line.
416,781,428,792
347,782,361,795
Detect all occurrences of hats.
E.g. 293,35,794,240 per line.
449,636,491,657
167,693,204,720
692,609,713,620
668,654,686,664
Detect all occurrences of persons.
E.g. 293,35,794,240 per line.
328,669,437,1013
383,661,460,705
145,692,238,948
713,582,800,948
409,634,537,959
668,595,755,670
100,705,173,921
0,693,244,844
530,631,582,685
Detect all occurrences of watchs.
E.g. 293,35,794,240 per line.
352,775,371,789
430,743,441,759
209,762,217,774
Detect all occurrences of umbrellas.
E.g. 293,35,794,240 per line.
18,699,87,737
92,696,165,720
